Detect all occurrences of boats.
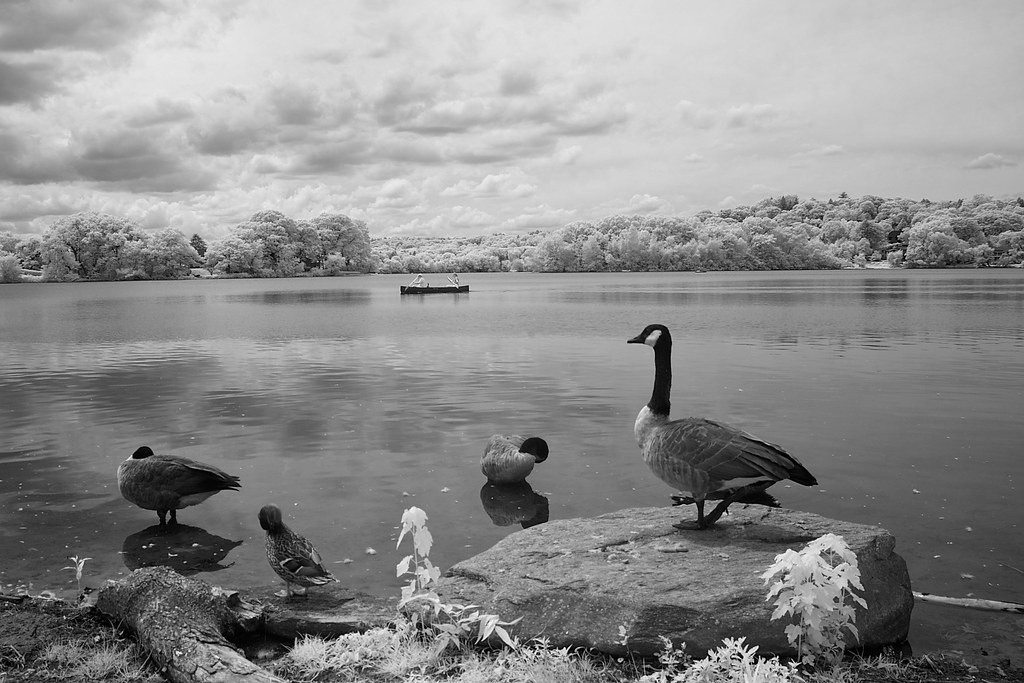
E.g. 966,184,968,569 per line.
400,285,469,295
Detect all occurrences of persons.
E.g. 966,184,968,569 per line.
413,274,426,288
450,273,459,286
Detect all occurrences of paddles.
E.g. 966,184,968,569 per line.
447,276,463,292
401,276,418,294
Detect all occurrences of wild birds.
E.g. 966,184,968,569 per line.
118,446,242,535
625,323,819,531
478,431,549,483
257,503,341,598
